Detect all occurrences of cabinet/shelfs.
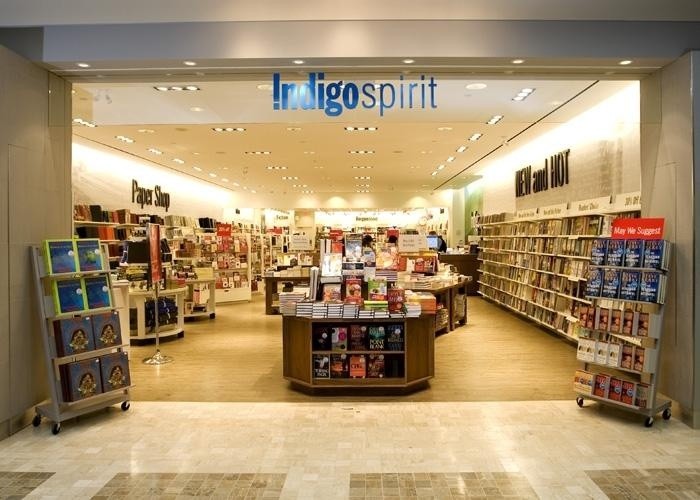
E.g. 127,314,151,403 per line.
30,243,136,416
282,303,437,390
573,237,673,412
75,220,289,282
192,232,252,303
477,210,641,345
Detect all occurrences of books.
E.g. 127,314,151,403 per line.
477,212,634,336
572,239,671,410
73,204,251,314
260,223,464,379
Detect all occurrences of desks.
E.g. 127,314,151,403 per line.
130,283,188,339
184,279,216,320
404,275,472,334
265,268,310,315
